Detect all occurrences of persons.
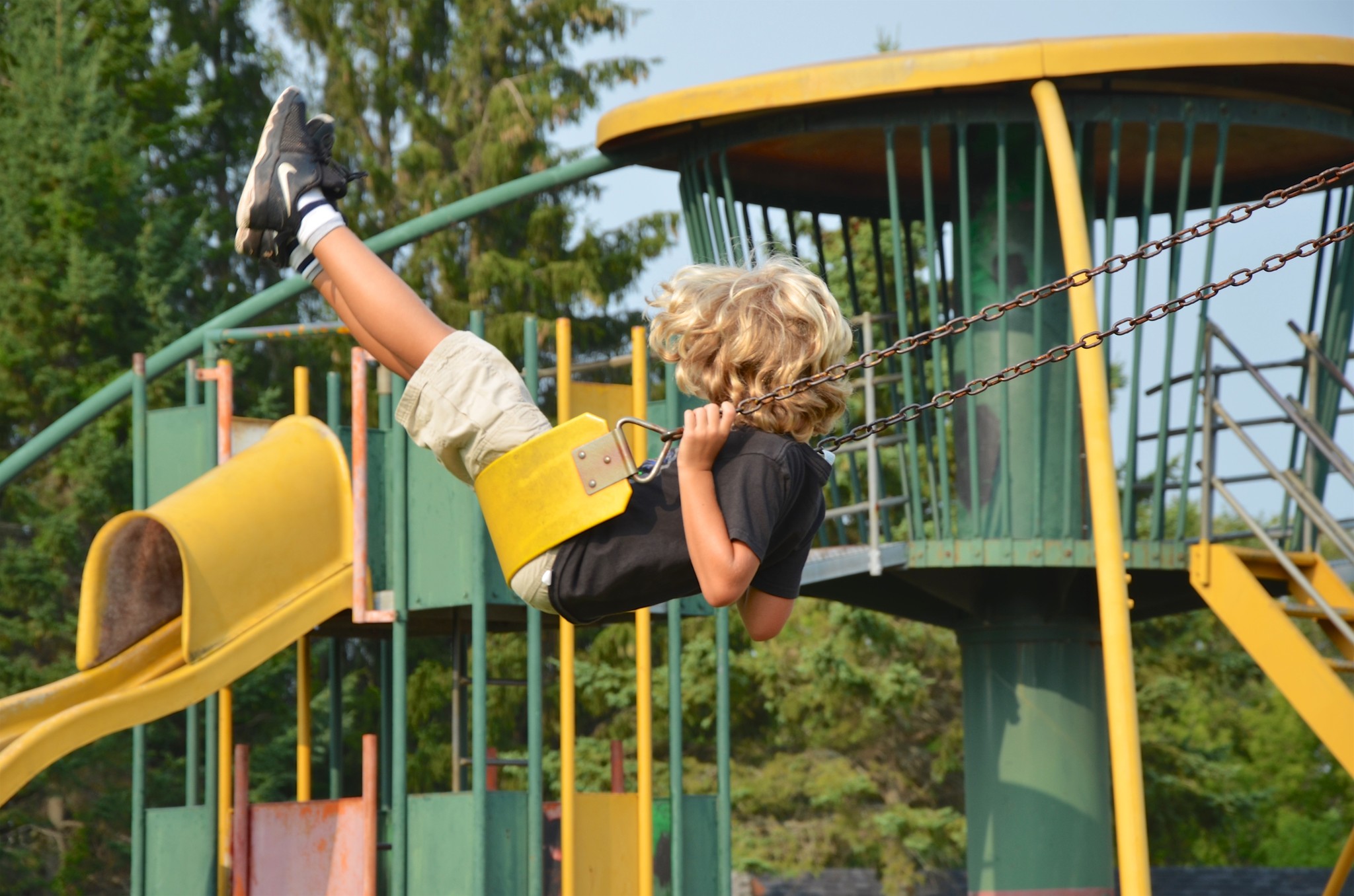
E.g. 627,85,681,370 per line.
234,84,854,642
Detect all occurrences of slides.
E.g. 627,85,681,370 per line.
0,614,187,808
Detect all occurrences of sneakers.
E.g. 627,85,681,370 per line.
237,87,368,233
234,113,335,268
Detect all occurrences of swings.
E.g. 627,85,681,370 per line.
467,164,1354,587
